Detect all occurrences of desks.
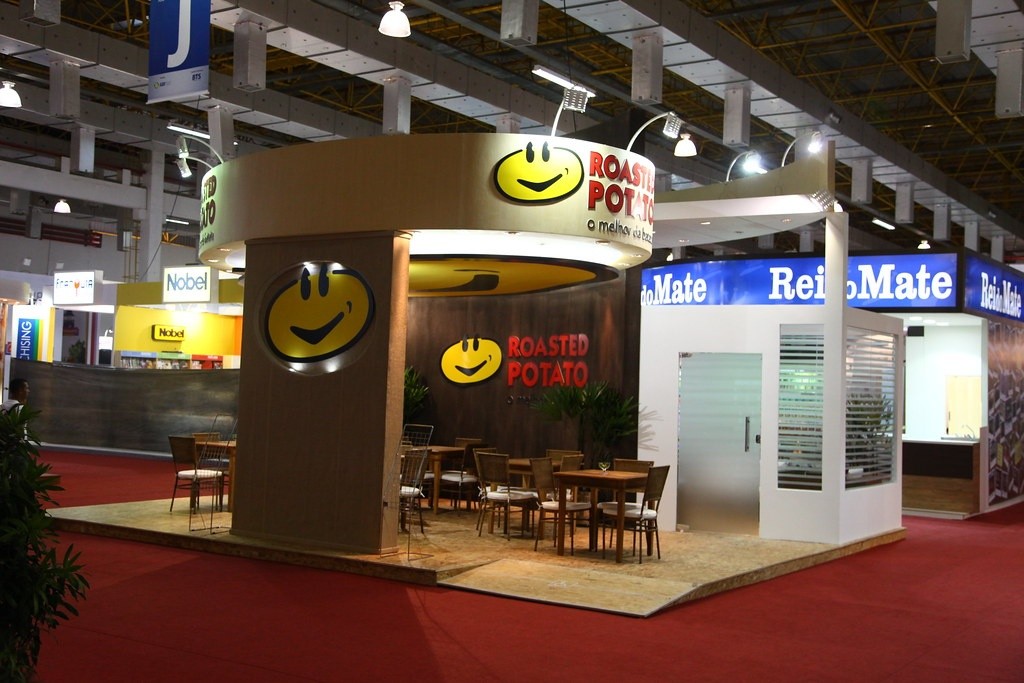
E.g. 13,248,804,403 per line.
410,447,466,514
192,441,236,512
553,470,655,563
508,457,582,539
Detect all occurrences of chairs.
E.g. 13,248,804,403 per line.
169,412,670,565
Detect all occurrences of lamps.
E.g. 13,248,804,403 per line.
54,198,71,213
166,122,211,140
0,79,22,107
550,87,588,135
177,135,224,164
674,133,697,157
177,157,213,177
378,2,410,37
627,111,683,152
531,0,596,98
917,239,931,249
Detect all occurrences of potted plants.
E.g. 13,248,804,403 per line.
528,379,642,527
